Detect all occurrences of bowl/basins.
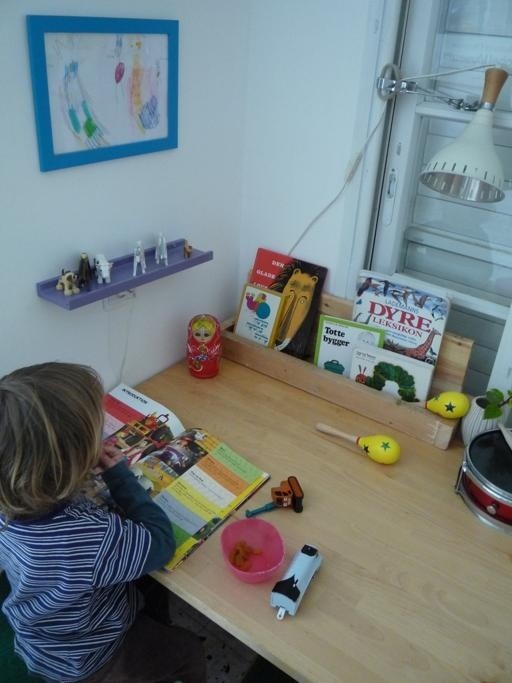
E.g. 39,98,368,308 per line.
220,518,285,582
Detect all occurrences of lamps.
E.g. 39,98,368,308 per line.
376,62,510,204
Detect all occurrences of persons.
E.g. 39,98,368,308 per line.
0,361,209,682
186,313,224,379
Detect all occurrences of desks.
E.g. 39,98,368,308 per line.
72,336,512,683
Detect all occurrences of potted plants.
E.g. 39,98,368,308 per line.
461,387,512,448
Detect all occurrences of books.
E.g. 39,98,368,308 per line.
248,247,329,359
231,284,286,348
349,340,436,404
314,313,386,375
72,380,272,574
351,268,451,365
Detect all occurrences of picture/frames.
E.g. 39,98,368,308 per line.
26,13,179,172
